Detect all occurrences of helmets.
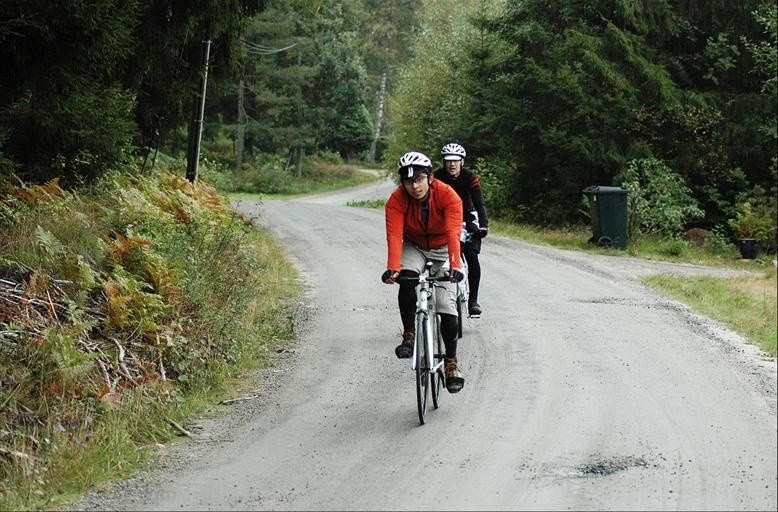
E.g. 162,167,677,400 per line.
441,143,466,158
397,151,432,173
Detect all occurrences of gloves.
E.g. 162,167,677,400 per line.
444,270,463,282
382,270,400,283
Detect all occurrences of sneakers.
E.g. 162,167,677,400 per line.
468,299,481,314
444,356,464,393
395,328,415,358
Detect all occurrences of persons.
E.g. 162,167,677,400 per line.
379,150,469,396
431,141,490,325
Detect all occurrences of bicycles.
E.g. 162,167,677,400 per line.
387,259,454,427
456,225,489,339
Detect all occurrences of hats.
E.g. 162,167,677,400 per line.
444,155,462,160
400,165,425,179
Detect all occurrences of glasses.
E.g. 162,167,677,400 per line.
401,175,425,185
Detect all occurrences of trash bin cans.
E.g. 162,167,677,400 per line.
582,184,630,250
